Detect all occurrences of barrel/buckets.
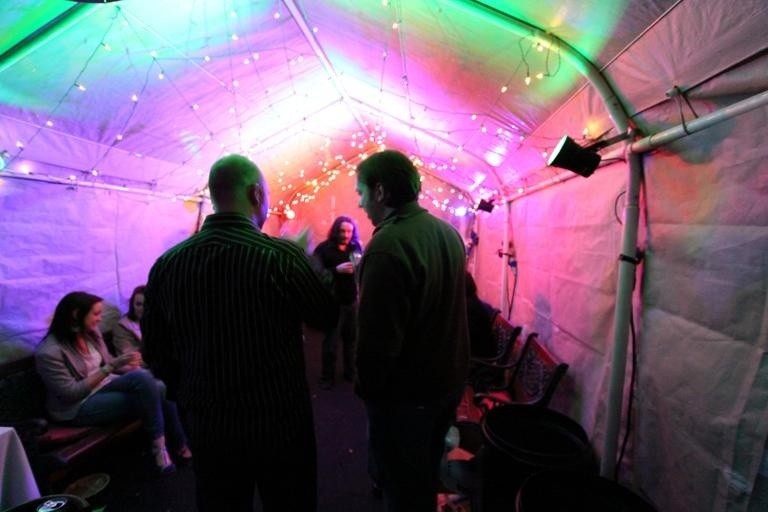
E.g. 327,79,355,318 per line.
479,406,591,512
65,473,113,505
0,494,90,512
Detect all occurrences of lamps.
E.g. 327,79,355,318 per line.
476,199,496,213
546,127,643,178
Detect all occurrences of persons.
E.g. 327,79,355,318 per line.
465,268,500,389
33,289,195,476
311,215,366,392
349,149,473,511
111,285,148,368
139,156,344,511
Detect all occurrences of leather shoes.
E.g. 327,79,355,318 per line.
177,442,193,464
152,446,176,477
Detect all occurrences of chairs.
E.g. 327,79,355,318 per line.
453,332,568,462
483,302,502,326
468,313,522,386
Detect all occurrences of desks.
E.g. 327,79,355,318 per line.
0,426,42,511
465,403,597,512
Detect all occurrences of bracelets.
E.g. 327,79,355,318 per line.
102,366,108,374
109,363,118,369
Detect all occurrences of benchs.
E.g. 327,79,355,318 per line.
0,330,146,478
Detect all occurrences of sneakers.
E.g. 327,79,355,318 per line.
320,374,335,391
